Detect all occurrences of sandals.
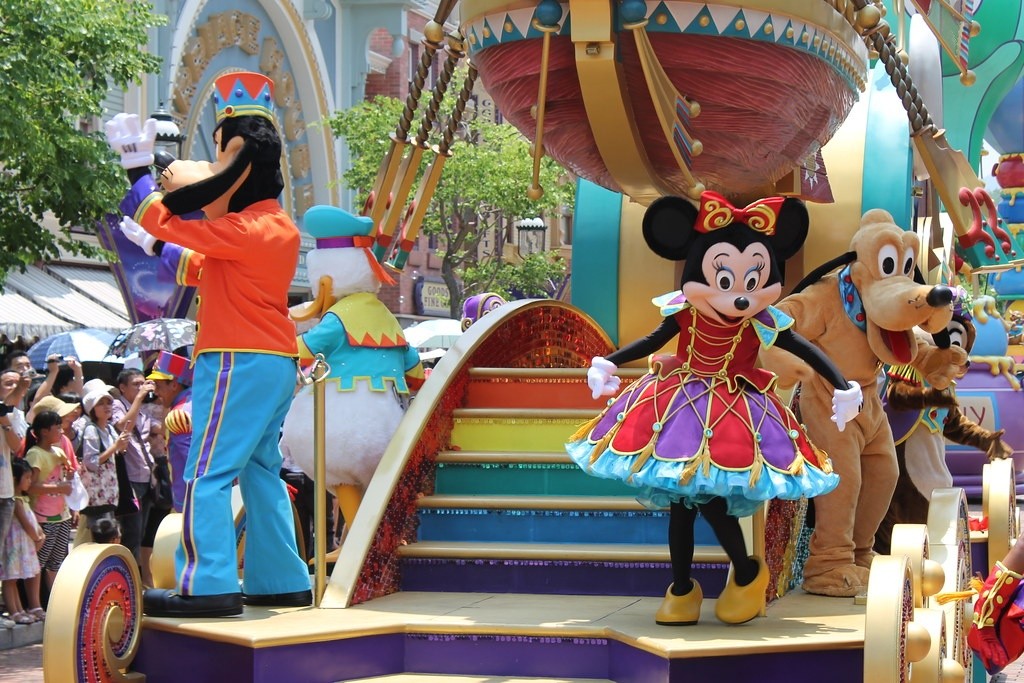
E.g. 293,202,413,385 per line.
20,610,40,621
12,612,33,624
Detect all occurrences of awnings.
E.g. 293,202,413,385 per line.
0,285,76,343
2,262,133,335
43,265,131,322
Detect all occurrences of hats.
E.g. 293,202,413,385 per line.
82,388,114,415
145,349,195,386
81,378,114,397
32,395,81,419
63,472,89,511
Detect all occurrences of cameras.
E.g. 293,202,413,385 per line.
22,368,36,378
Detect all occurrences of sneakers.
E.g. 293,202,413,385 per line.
0,616,15,628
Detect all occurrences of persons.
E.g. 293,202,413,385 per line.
0,350,131,629
967,531,1024,676
146,350,236,514
112,367,170,580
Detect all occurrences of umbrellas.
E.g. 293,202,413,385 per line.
403,319,462,348
26,328,139,371
100,304,196,365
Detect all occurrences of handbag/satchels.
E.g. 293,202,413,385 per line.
105,425,140,515
149,463,161,500
78,424,119,512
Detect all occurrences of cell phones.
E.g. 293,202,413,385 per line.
63,358,75,361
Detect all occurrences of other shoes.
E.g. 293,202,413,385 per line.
27,607,46,620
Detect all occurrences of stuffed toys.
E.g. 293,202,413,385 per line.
758,208,968,598
870,285,1014,556
565,191,863,625
279,205,425,571
105,69,313,618
